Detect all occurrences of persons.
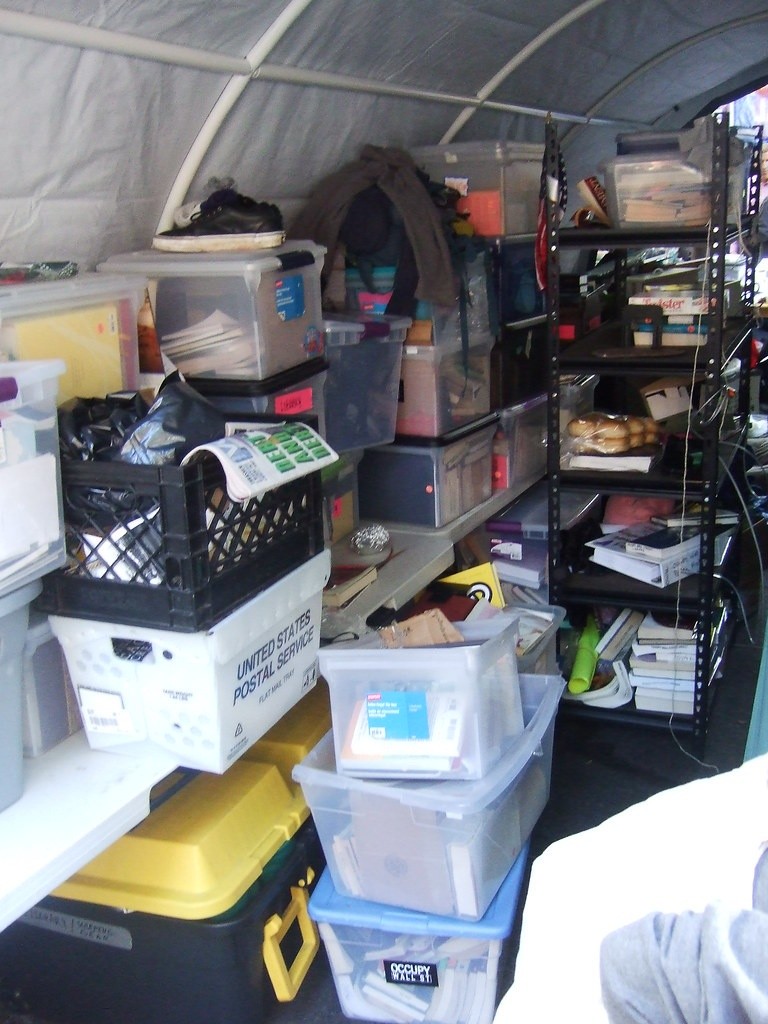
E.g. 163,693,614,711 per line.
728,143,768,416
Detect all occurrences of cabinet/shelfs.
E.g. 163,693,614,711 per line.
536,112,765,746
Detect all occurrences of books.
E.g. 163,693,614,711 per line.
1,168,730,920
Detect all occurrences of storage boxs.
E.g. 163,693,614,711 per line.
2,123,749,1024
